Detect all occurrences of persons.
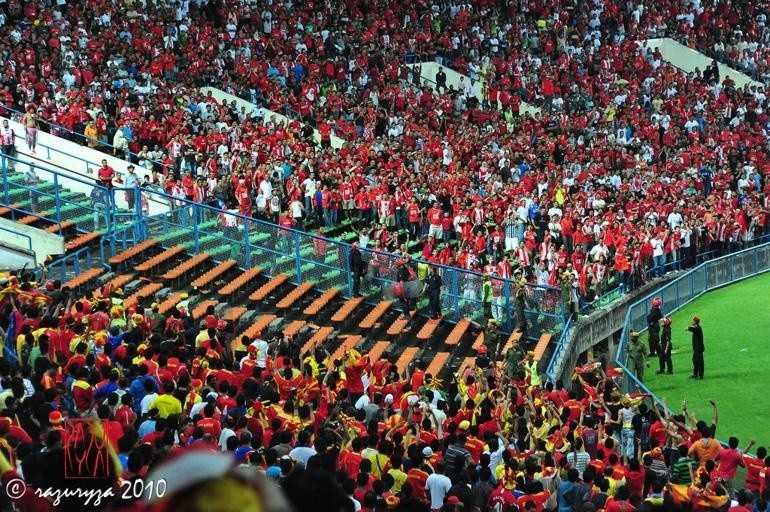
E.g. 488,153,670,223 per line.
0,0,770,512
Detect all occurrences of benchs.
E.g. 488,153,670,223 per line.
2,168,554,385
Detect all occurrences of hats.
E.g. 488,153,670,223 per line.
396,257,406,265
0,275,755,512
352,241,358,248
